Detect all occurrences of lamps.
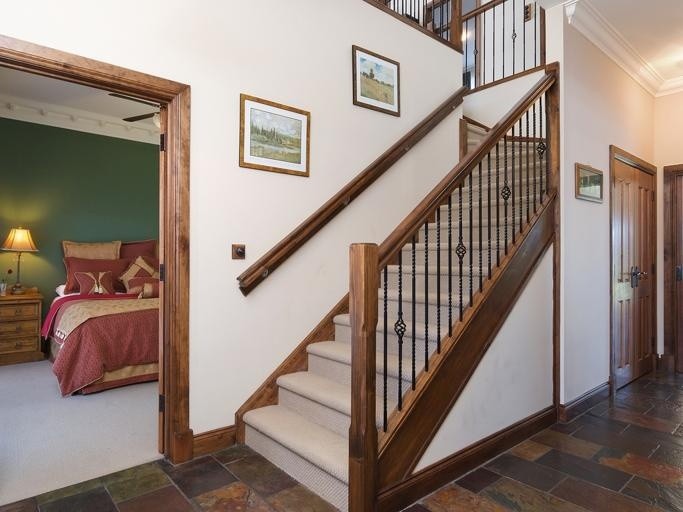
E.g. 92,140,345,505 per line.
0,225,38,295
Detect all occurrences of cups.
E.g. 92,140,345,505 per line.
0,282,8,296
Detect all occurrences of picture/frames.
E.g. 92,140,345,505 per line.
239,92,310,178
352,45,401,116
573,164,604,202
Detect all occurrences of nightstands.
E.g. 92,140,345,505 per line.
1,288,45,366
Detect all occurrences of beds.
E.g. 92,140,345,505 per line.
44,297,159,394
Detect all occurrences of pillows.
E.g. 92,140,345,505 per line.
59,241,160,297
